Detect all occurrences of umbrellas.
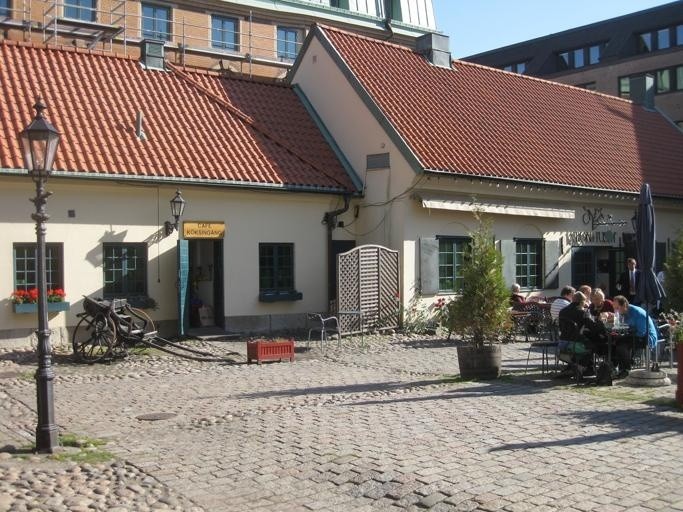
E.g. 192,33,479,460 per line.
629,183,666,369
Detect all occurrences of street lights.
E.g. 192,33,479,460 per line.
14,91,66,456
629,207,637,233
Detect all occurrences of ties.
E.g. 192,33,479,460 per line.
631,271,635,293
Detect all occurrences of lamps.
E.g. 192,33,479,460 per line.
164,187,186,236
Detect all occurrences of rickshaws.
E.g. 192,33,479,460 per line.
68,293,239,369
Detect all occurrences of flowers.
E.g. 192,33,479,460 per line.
10,288,65,303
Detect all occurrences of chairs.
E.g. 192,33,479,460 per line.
304,311,341,356
508,295,675,387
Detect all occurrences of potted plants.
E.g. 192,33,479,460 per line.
440,193,514,380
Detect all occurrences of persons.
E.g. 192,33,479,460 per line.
652,262,668,307
542,282,658,384
508,281,531,311
616,257,644,305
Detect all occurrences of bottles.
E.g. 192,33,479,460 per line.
613,310,620,326
620,312,625,326
